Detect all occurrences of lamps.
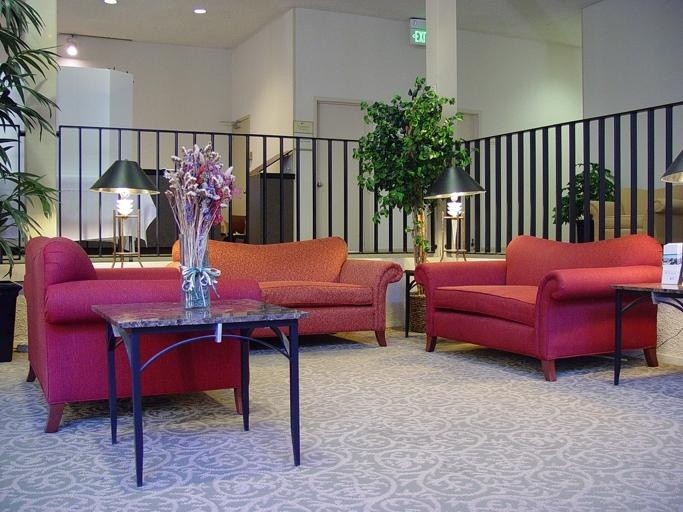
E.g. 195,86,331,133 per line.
659,153,683,185
421,164,488,263
89,159,161,271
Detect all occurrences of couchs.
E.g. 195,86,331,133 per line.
414,234,664,383
165,236,404,347
23,236,263,434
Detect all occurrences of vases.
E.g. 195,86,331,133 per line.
178,233,222,310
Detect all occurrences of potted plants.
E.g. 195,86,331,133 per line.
0,0,65,364
552,160,615,242
351,71,481,333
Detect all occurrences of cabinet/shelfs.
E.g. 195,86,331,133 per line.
0,65,134,248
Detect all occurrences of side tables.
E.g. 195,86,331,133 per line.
89,296,311,488
405,269,417,339
609,282,683,386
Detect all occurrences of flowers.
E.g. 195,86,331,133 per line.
162,140,244,311
